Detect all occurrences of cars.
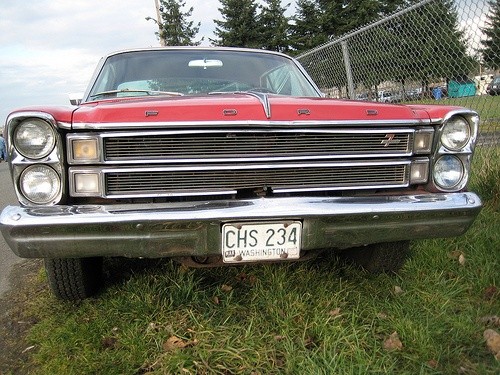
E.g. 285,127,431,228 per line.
0,46,482,300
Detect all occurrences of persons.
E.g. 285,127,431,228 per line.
0,134,9,163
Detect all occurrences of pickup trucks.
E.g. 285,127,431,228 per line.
372,91,399,102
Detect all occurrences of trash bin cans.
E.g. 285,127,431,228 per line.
447,78,476,98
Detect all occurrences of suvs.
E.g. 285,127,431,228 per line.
486,77,500,96
